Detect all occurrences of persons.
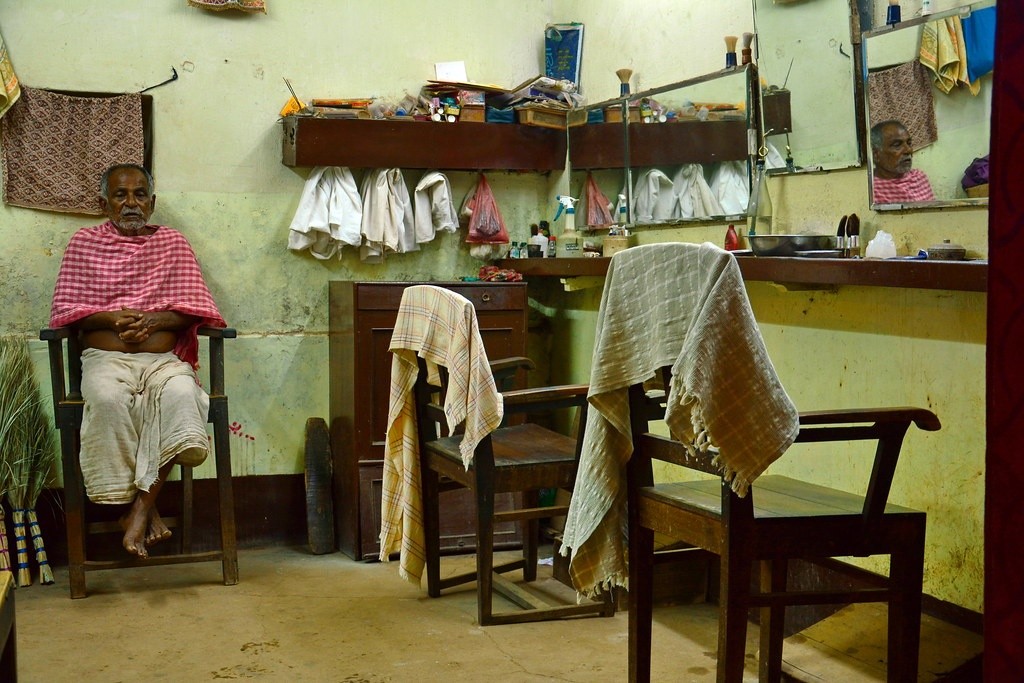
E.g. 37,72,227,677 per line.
869,121,937,204
51,163,228,560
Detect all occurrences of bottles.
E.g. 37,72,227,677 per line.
725,223,738,251
510,242,519,259
519,242,529,259
548,236,557,257
747,159,773,235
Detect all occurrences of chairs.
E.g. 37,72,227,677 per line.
37,322,241,600
401,286,618,627
610,243,944,683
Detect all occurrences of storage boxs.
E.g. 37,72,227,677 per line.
457,89,487,123
605,104,642,124
516,107,566,130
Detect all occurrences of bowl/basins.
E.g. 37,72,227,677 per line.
528,244,543,258
928,239,966,261
743,234,835,256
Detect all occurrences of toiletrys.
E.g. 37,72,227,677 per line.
553,195,584,258
724,223,740,250
617,194,628,222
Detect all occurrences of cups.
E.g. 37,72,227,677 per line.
886,5,901,24
921,0,934,16
726,53,737,68
621,83,630,97
742,49,751,64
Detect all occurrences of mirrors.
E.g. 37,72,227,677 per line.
566,62,757,233
861,0,997,211
752,0,864,177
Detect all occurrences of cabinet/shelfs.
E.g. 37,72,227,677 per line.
328,278,530,565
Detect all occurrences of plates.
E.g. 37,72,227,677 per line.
730,249,753,256
794,250,843,258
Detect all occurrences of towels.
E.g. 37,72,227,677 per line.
48,220,227,371
632,166,674,222
708,160,750,216
359,168,421,264
960,5,996,85
674,163,724,218
764,139,786,168
868,56,939,153
556,240,800,606
413,169,461,243
377,286,504,591
285,166,362,266
1,83,145,217
919,14,981,96
873,169,936,204
0,31,21,121
